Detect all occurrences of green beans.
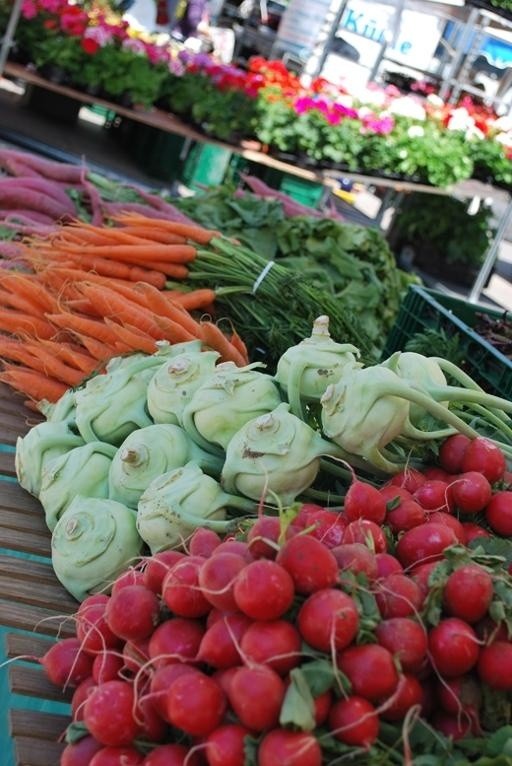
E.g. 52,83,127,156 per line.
472,310,511,366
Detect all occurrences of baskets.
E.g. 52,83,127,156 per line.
377,283,512,403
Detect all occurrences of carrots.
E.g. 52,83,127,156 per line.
1,146,347,409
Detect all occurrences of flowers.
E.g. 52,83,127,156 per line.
1,0,510,188
